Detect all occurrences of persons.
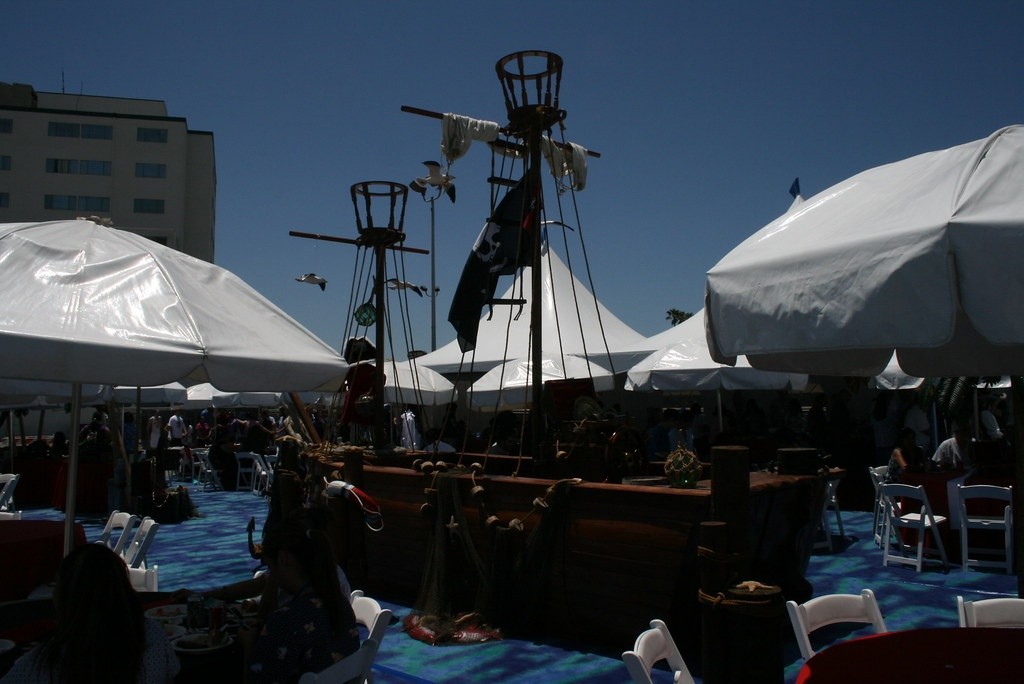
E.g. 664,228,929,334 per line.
19,393,832,488
170,509,351,617
931,422,976,469
0,544,181,684
883,428,927,502
238,522,360,683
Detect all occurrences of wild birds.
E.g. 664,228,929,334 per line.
417,161,457,204
384,278,423,298
294,273,326,291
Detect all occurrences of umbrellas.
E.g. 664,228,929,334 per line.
0,216,349,594
0,307,1014,407
706,123,1022,378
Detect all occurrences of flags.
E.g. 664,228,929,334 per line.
448,167,547,354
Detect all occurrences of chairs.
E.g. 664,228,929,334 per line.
956,594,1024,628
787,587,889,662
121,514,160,592
0,471,20,519
877,482,951,574
187,434,282,499
867,470,903,549
622,618,695,684
347,589,383,649
868,465,892,482
956,483,1015,575
94,508,138,560
299,605,392,684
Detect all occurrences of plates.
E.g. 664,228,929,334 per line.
0,639,15,651
144,602,234,654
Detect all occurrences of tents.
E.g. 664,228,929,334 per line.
400,242,665,381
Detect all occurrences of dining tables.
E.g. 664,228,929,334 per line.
1,590,335,683
795,625,1024,683
0,517,89,599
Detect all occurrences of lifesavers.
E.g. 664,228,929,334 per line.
326,480,381,533
